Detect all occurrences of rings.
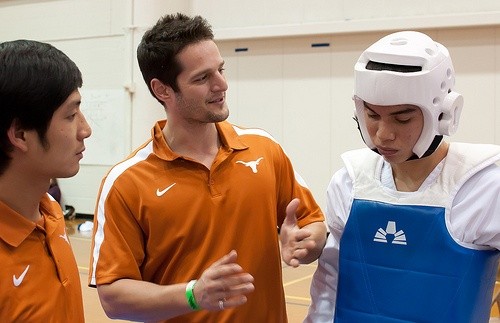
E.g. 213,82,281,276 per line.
217,301,226,309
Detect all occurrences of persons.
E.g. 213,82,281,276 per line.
89,13,326,323
0,39,92,323
301,31,500,323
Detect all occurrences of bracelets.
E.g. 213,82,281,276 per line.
186,279,201,314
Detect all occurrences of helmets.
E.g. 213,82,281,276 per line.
352,30,464,158
